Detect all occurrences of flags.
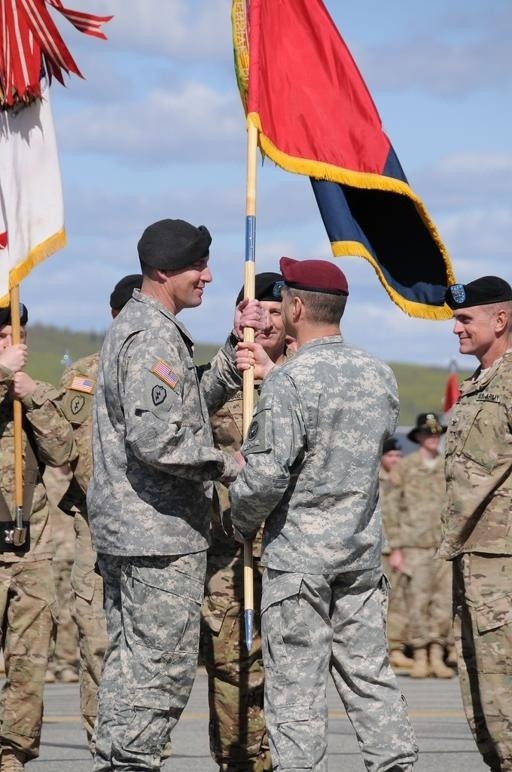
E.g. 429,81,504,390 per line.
228,1,457,319
440,373,461,414
0,73,68,300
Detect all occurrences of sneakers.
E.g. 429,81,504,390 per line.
0,748,25,772
44,659,79,683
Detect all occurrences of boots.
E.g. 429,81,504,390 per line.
388,643,458,679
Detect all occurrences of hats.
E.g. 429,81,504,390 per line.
110,219,348,316
382,438,400,453
0,302,28,325
445,276,512,310
408,413,448,443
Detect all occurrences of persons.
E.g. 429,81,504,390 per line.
223,253,423,772
39,463,80,685
192,270,302,770
60,273,147,761
369,436,417,677
0,303,80,772
439,274,510,772
379,411,458,681
86,219,268,771
